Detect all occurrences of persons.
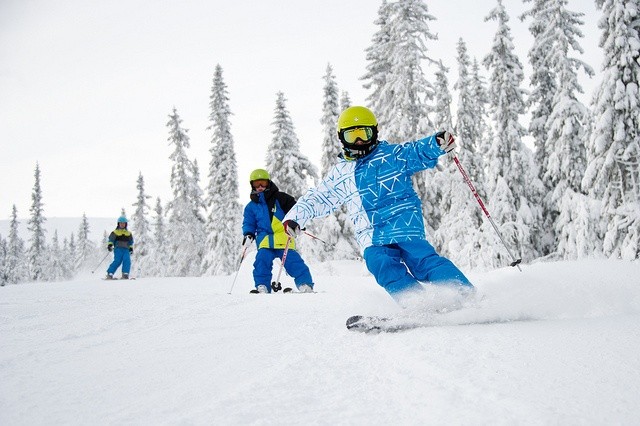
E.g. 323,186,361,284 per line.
281,104,488,309
242,168,315,294
106,216,134,281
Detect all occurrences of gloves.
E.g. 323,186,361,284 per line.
129,247,133,254
107,244,112,251
436,130,456,152
242,235,253,246
284,219,301,238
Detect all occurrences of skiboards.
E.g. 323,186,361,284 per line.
346,313,543,333
258,285,314,296
107,277,137,281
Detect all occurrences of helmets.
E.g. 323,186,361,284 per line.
118,217,128,222
250,169,270,180
338,106,378,159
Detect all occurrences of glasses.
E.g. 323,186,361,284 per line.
340,127,377,144
252,181,268,187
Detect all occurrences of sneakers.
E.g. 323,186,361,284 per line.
107,273,112,278
122,274,128,279
